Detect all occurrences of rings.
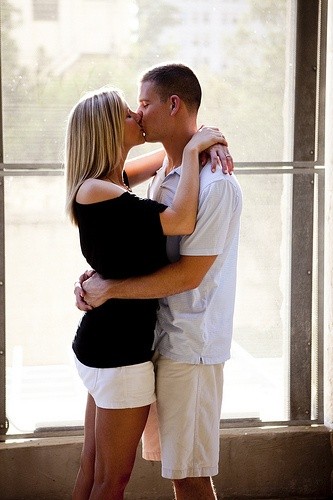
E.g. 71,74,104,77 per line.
225,154,231,158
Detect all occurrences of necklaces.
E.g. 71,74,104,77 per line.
108,176,133,194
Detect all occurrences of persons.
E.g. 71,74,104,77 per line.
76,62,240,499
65,89,234,500
